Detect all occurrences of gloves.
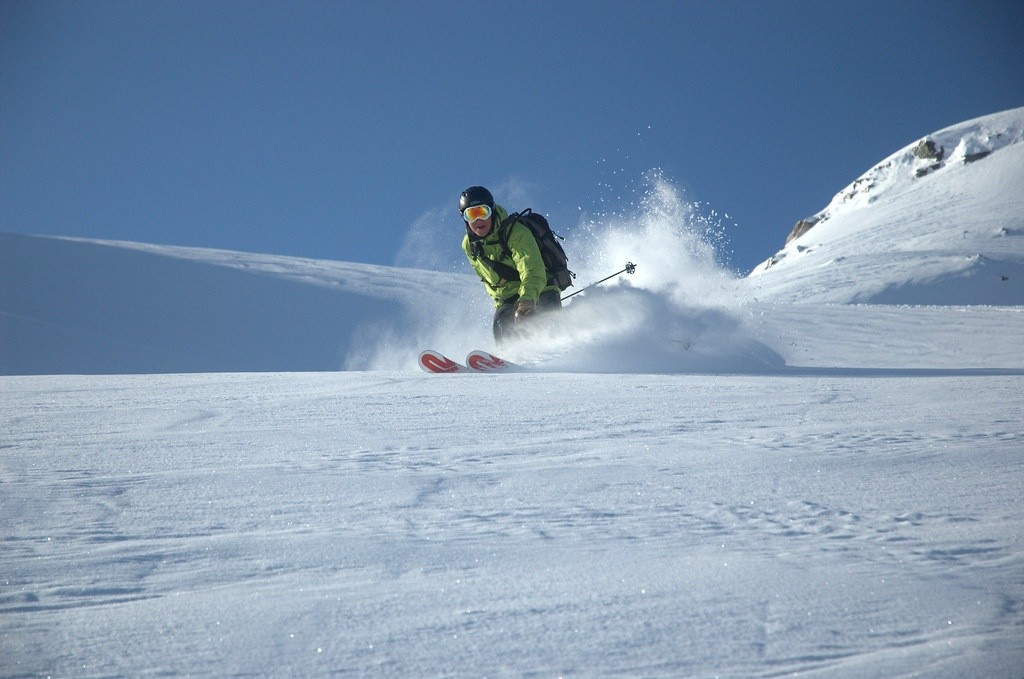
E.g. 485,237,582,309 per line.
515,301,536,322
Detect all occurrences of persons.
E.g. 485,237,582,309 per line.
459,185,582,371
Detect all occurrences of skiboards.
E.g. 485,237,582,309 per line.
417,348,522,373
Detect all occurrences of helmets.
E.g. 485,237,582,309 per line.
459,186,495,214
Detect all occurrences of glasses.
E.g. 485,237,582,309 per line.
464,205,492,223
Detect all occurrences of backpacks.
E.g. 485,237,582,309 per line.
467,212,576,292
